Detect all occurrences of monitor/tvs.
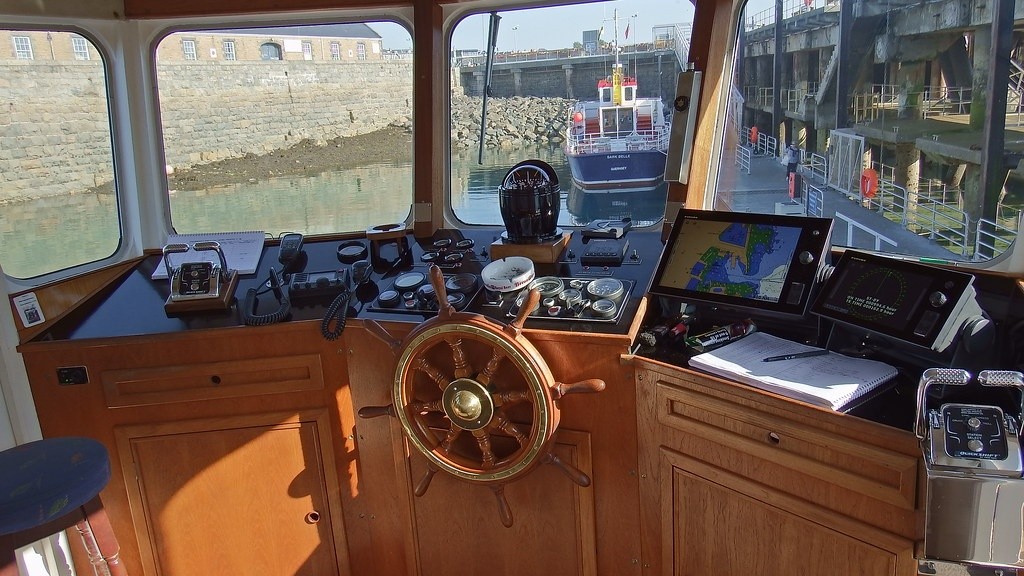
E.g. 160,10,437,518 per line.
810,249,975,351
648,208,835,316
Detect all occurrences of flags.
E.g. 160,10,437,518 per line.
624,23,630,39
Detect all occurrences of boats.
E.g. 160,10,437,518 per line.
564,8,674,191
562,181,668,227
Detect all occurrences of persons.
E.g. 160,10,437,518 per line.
784,140,800,182
750,123,759,150
572,125,584,153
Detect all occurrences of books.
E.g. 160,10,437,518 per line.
687,331,899,415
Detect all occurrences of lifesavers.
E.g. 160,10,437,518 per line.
750,126,758,143
861,169,877,198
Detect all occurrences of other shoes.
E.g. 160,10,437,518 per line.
786,176,788,182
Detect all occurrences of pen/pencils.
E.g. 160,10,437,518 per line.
763,348,830,362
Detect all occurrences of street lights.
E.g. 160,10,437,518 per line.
512,25,518,63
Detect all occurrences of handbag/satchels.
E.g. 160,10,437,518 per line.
781,154,789,166
